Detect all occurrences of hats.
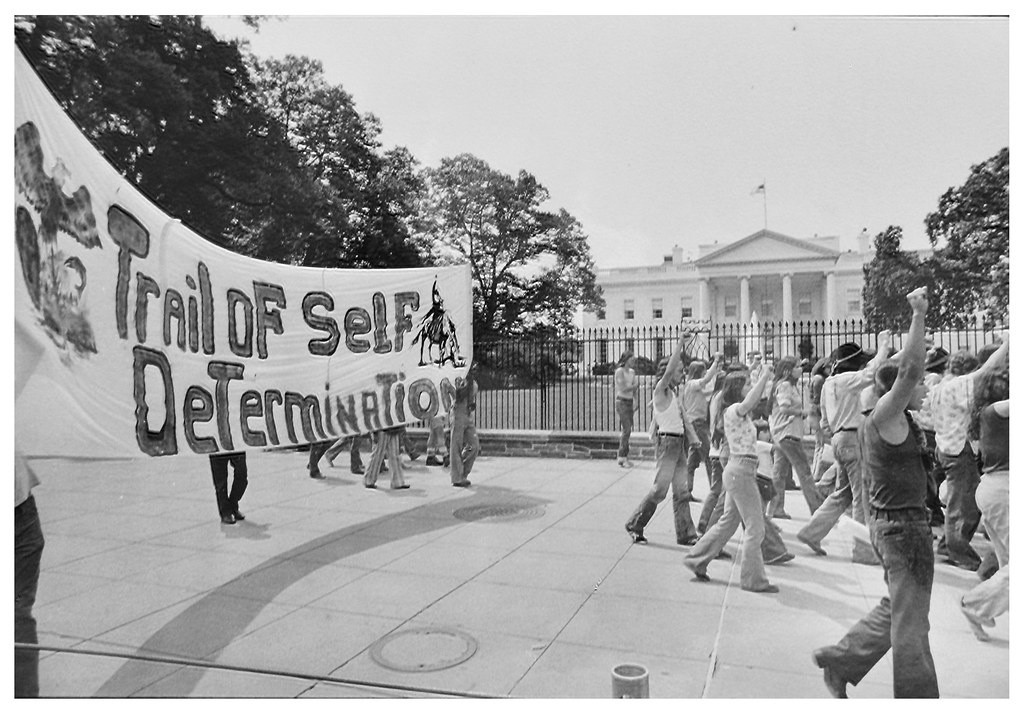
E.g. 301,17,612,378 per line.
950,350,980,374
811,358,830,374
979,343,1007,370
832,342,870,369
924,346,949,370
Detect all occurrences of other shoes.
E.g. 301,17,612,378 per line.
619,458,633,467
716,549,732,561
799,535,826,555
964,608,992,642
774,512,792,519
814,649,848,699
690,495,702,503
383,466,388,471
395,484,410,489
352,469,364,474
401,463,412,470
365,483,377,489
311,472,326,479
410,453,420,461
325,452,334,466
778,553,795,562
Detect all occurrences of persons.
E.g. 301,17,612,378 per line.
614,352,640,468
14,459,45,699
306,417,450,490
451,360,480,487
209,452,249,525
625,285,1009,698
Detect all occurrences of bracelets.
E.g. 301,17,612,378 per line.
912,313,926,319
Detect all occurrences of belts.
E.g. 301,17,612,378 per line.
657,432,684,438
616,396,633,402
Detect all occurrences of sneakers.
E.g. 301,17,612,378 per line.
625,523,648,544
692,569,710,583
677,537,700,545
444,455,451,467
763,583,779,593
453,479,471,487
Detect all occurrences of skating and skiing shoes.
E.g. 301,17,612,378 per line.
426,456,443,466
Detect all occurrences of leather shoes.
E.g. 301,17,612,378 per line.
221,510,245,524
